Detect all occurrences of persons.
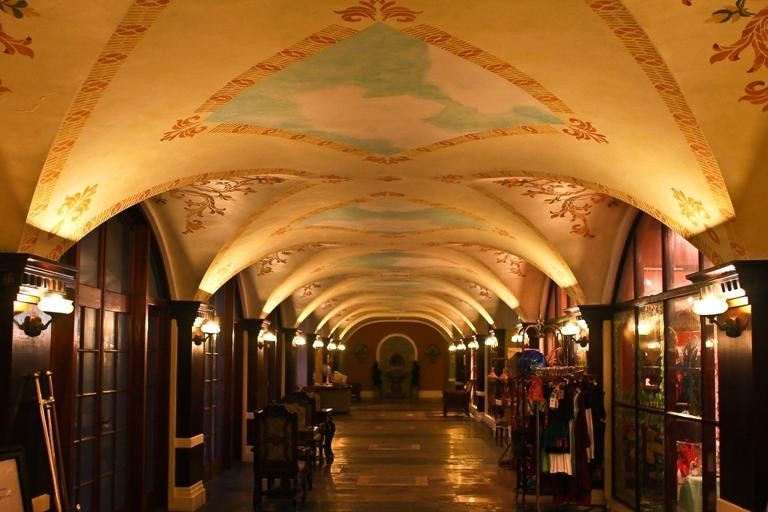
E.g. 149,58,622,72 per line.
370,361,385,398
408,361,422,399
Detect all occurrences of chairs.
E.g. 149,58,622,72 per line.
442,379,474,417
251,390,327,511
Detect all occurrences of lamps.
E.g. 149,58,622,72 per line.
691,296,742,338
22,291,73,339
559,319,588,347
193,319,221,346
